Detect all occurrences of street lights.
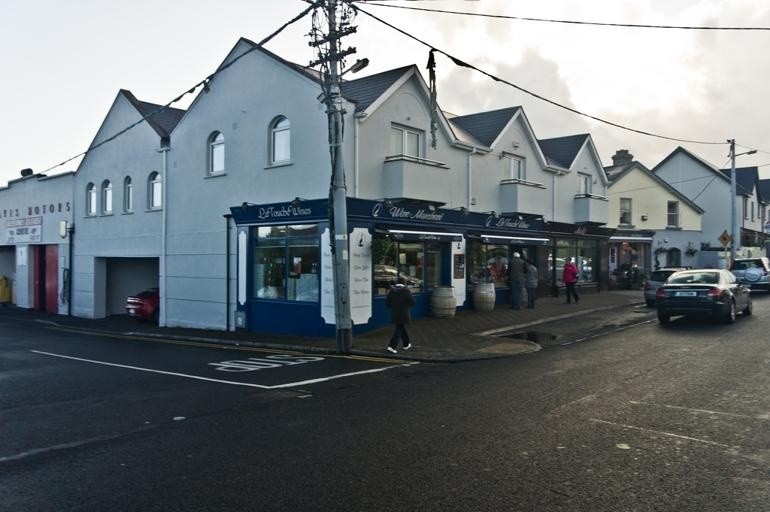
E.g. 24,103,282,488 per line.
329,58,369,353
727,139,757,256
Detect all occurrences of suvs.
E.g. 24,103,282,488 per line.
729,257,770,291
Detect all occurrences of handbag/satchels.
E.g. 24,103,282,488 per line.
572,272,580,279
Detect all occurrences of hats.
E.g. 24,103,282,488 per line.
513,251,520,257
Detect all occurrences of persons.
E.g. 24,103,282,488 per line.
387,277,416,354
507,252,539,309
563,257,581,304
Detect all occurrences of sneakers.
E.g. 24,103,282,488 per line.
402,343,412,350
510,305,535,309
563,302,572,305
387,346,397,353
573,297,580,305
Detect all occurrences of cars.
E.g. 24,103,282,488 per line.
644,267,752,325
374,264,424,295
548,256,592,281
125,287,159,324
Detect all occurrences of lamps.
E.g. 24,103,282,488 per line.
58,219,75,240
